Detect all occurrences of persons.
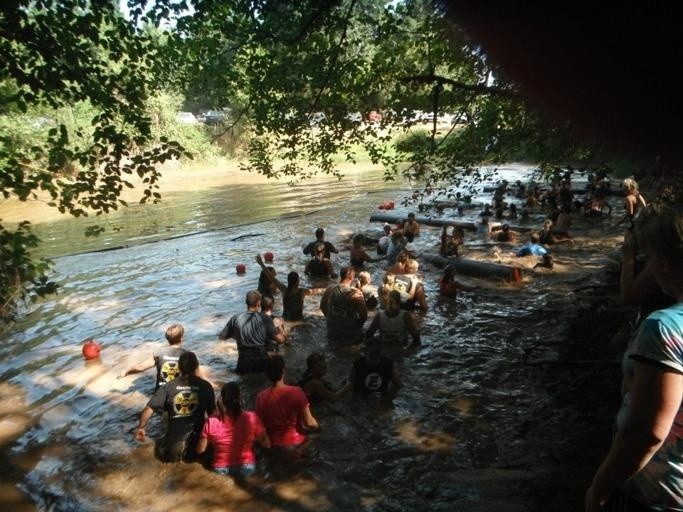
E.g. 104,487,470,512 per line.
134,352,216,462
621,229,678,323
439,177,647,299
254,356,321,460
585,198,683,512
131,213,428,407
194,382,271,476
